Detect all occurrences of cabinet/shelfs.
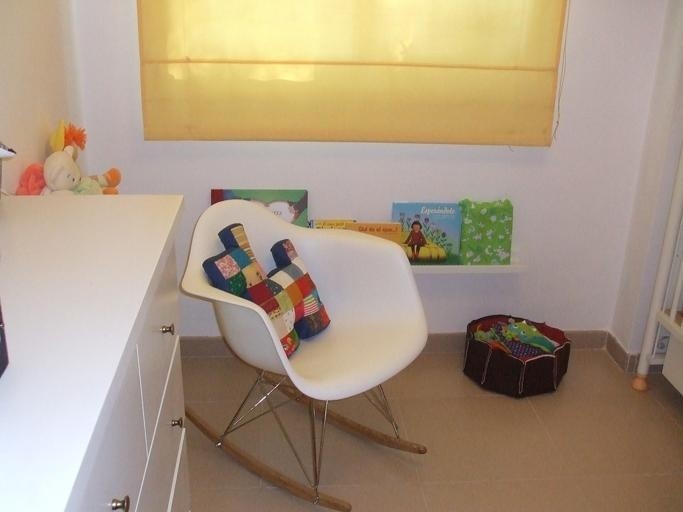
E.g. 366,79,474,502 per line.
0,195,184,512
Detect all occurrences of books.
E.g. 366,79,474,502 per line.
209,188,312,231
389,202,463,266
308,218,361,233
344,220,403,246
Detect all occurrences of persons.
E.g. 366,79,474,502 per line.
403,221,426,261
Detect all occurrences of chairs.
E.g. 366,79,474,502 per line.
179,198,428,512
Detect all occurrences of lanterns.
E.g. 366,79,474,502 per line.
12,163,46,195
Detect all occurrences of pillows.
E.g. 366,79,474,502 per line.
203,224,330,358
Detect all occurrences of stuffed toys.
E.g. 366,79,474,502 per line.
39,145,121,196
